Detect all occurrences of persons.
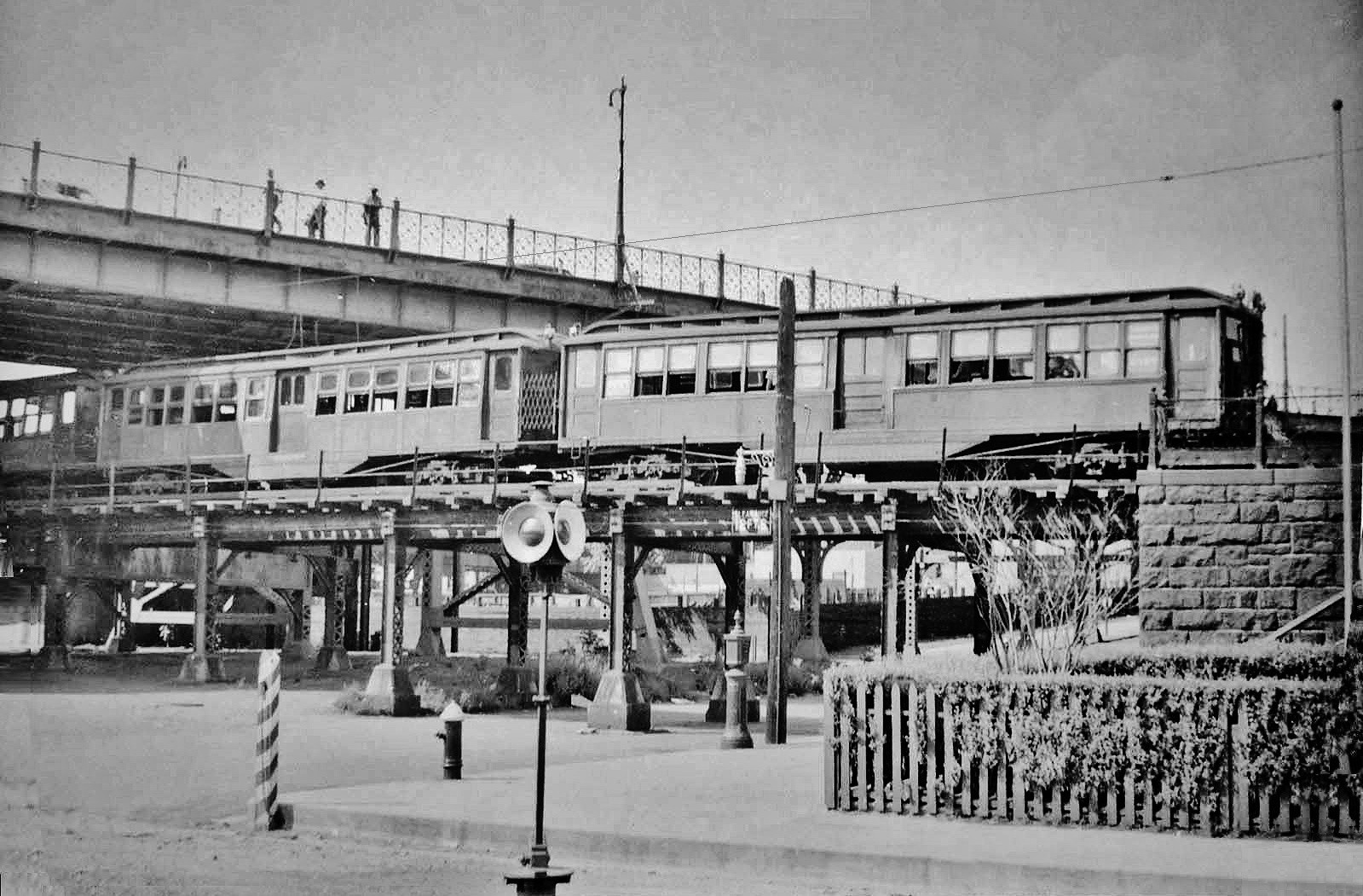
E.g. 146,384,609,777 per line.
362,187,383,246
1048,357,1079,379
950,360,970,381
765,370,778,394
304,179,329,239
262,175,284,231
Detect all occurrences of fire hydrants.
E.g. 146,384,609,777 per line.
436,701,465,781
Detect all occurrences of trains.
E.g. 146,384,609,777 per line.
1,284,1268,503
